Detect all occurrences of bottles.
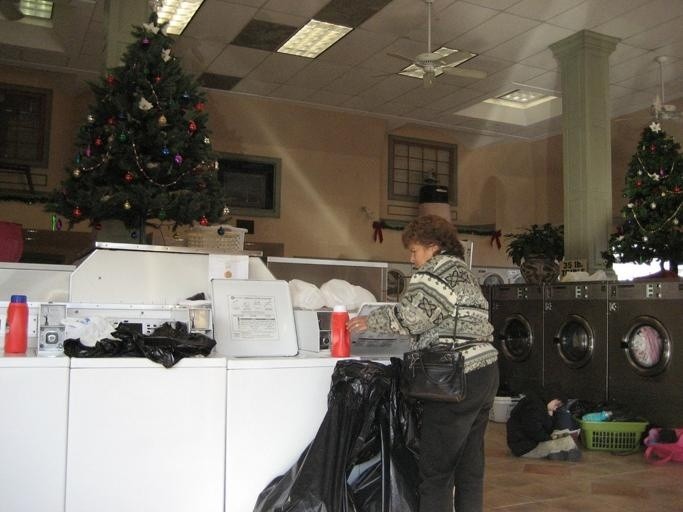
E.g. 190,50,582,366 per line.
581,410,612,422
330,304,351,358
3,294,28,353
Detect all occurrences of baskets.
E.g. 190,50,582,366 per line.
572,409,650,453
184,224,250,252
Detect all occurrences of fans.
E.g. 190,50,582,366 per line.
613,56,681,123
369,2,488,80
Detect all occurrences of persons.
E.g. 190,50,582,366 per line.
344,215,501,512
507,385,582,461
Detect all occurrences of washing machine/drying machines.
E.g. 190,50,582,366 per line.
1,241,414,511
388,263,683,430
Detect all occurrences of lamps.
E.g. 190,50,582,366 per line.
421,71,435,91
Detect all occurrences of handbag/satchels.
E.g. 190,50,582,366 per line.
643,427,683,466
399,346,467,403
